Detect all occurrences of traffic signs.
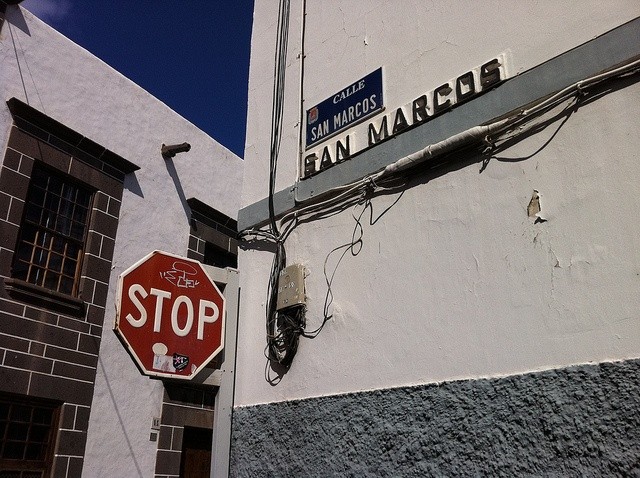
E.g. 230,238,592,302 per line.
114,249,226,381
